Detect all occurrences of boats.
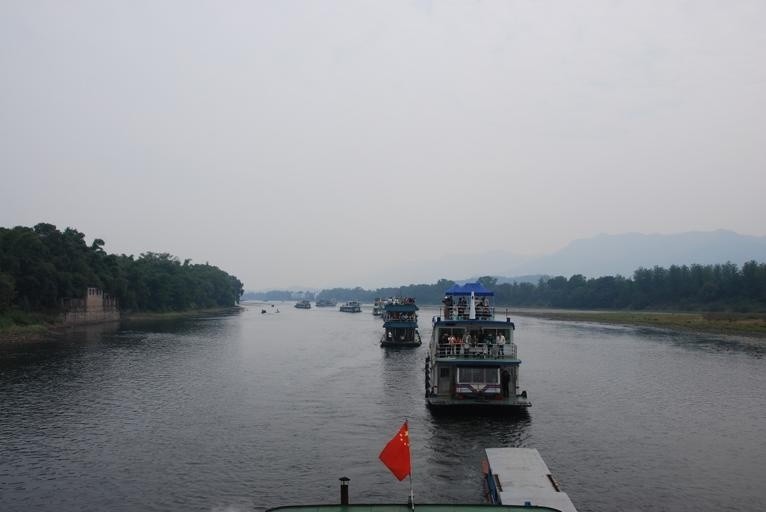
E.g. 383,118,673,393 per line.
294,297,337,309
339,300,362,314
371,295,423,347
423,282,532,409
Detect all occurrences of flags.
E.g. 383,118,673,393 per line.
378,420,411,481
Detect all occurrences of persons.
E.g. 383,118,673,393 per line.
437,288,507,358
382,294,419,342
